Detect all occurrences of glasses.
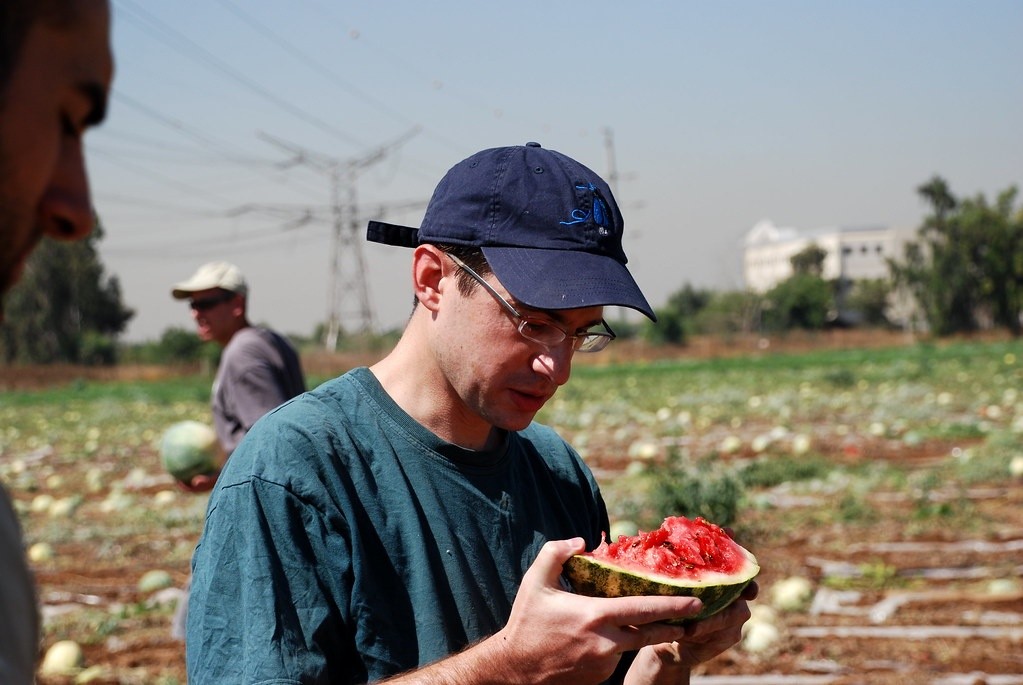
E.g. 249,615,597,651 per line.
439,246,616,354
189,290,235,312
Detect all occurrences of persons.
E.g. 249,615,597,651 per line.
172,260,309,494
186,141,759,685
0,0,115,684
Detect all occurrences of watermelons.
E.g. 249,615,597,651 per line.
611,520,637,544
162,420,222,484
562,515,760,624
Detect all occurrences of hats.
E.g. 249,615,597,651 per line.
173,260,248,303
365,142,659,325
